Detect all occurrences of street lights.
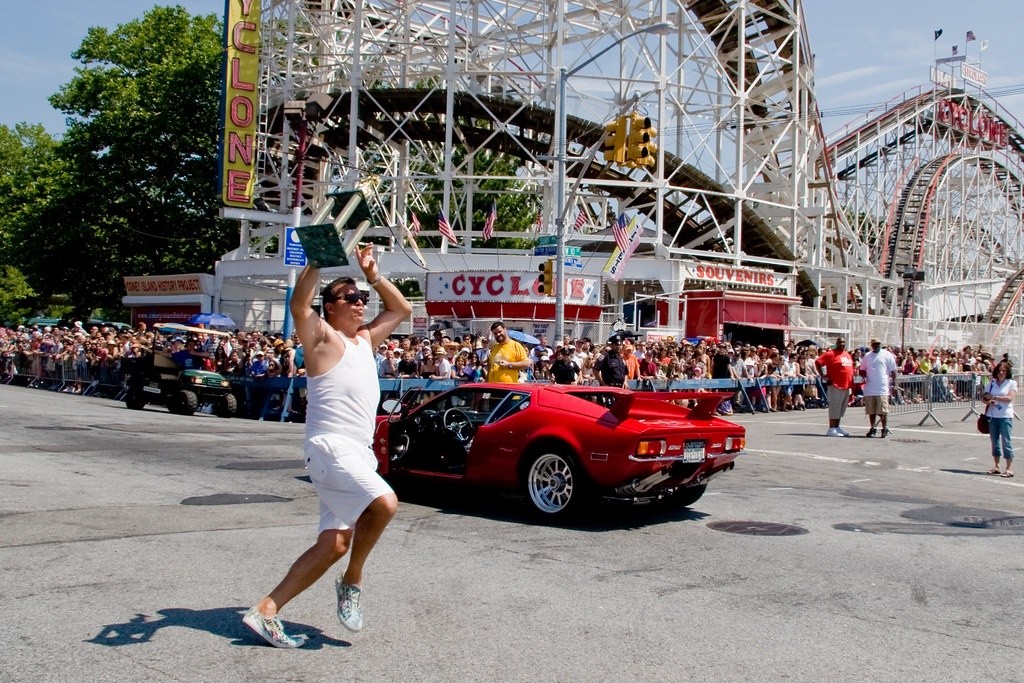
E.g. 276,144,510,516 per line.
283,93,334,337
555,22,676,342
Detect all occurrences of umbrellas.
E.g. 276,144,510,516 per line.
611,330,643,337
428,320,464,331
187,312,236,330
797,339,820,347
506,330,541,345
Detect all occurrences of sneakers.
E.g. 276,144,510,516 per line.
827,429,844,437
243,607,304,647
836,427,849,436
335,573,363,632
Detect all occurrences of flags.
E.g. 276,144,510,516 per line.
483,199,497,243
438,204,457,244
536,202,542,234
407,207,420,236
574,209,588,232
952,45,958,57
602,216,641,282
396,210,427,268
934,29,943,40
981,40,988,50
967,31,976,42
612,212,630,251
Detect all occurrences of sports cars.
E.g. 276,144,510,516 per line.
373,382,747,525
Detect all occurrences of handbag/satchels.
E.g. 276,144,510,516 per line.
978,414,989,433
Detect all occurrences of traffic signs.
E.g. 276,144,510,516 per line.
534,236,582,268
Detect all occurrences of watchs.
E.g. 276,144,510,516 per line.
509,362,513,369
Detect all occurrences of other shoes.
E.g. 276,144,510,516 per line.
987,469,1001,474
882,429,888,437
866,429,877,437
1001,471,1014,477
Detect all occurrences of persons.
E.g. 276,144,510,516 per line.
0,316,1013,418
484,321,530,423
981,362,1017,478
240,241,397,648
815,336,856,437
593,334,629,409
859,338,897,438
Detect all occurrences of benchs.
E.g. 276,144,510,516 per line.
153,353,180,383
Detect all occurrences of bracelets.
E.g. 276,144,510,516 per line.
367,274,382,286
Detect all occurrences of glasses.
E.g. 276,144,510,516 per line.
327,294,367,305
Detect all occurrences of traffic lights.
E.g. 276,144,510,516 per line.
645,127,657,167
604,115,627,164
538,262,552,294
626,112,651,168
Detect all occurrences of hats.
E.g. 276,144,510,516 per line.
555,342,562,347
534,346,545,351
561,347,570,356
18,320,140,348
568,345,575,350
257,350,264,356
871,339,881,345
609,335,624,344
171,336,186,344
273,339,284,347
379,338,490,361
540,356,550,362
